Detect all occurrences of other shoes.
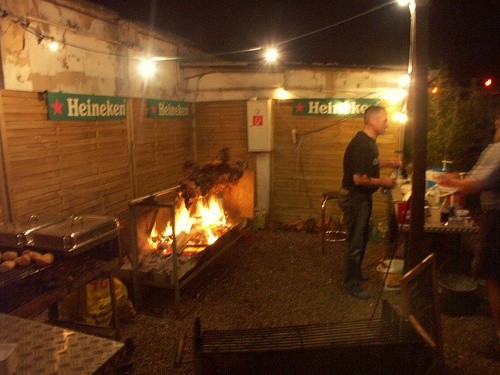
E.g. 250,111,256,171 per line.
344,273,370,299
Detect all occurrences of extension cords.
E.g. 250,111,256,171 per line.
292,129,298,144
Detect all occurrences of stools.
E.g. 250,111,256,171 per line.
320,192,347,243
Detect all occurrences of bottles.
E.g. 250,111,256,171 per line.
382,166,400,195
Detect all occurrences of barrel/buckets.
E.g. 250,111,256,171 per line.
437,276,477,317
377,259,403,290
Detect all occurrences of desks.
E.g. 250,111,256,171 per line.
390,187,480,274
0,312,125,375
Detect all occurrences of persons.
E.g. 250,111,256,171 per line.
435,117,500,275
336,105,397,299
